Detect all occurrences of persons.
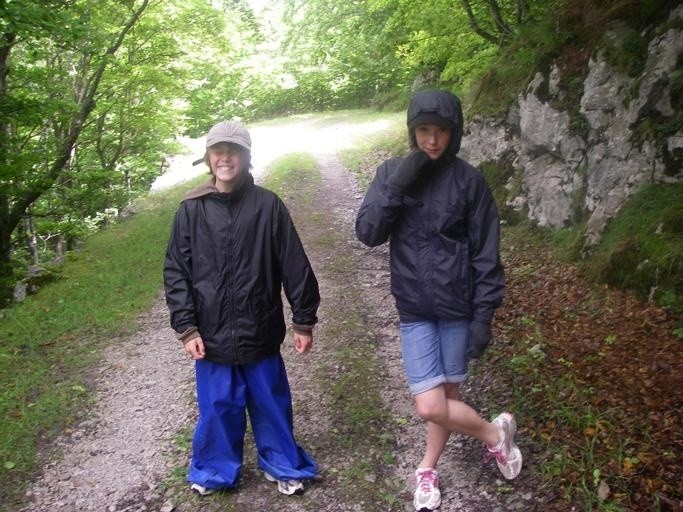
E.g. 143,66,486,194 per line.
354,90,523,512
161,121,322,497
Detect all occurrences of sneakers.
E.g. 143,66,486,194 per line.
413,465,442,512
264,471,305,496
191,482,215,496
487,412,523,480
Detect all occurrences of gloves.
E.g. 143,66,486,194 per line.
467,320,492,360
388,149,429,198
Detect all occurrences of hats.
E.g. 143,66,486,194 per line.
409,111,453,130
206,121,252,151
